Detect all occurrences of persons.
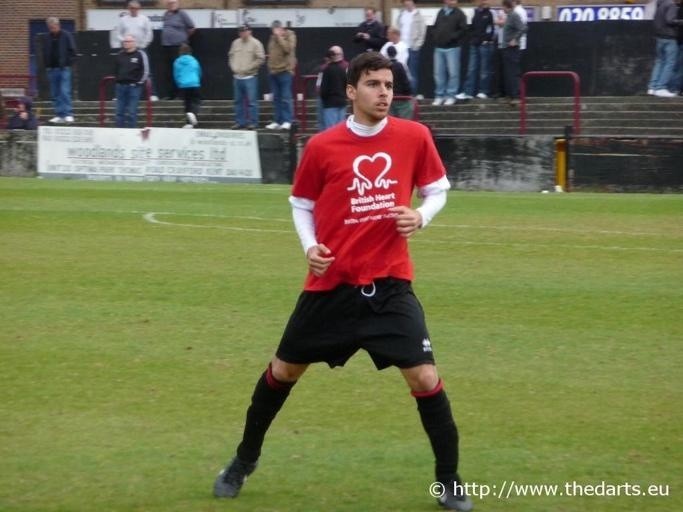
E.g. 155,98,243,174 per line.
39,16,80,124
113,33,150,128
227,24,265,130
264,20,298,130
646,0,682,97
170,43,203,129
157,0,195,99
207,51,479,510
110,0,159,102
314,1,530,131
7,97,36,129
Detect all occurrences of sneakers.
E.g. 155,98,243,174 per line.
647,89,653,95
264,121,279,129
432,92,487,105
279,121,291,129
652,88,677,97
186,112,197,125
64,116,73,122
149,95,158,101
436,477,472,512
213,456,260,499
47,116,63,123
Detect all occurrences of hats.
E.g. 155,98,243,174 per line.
237,23,250,31
